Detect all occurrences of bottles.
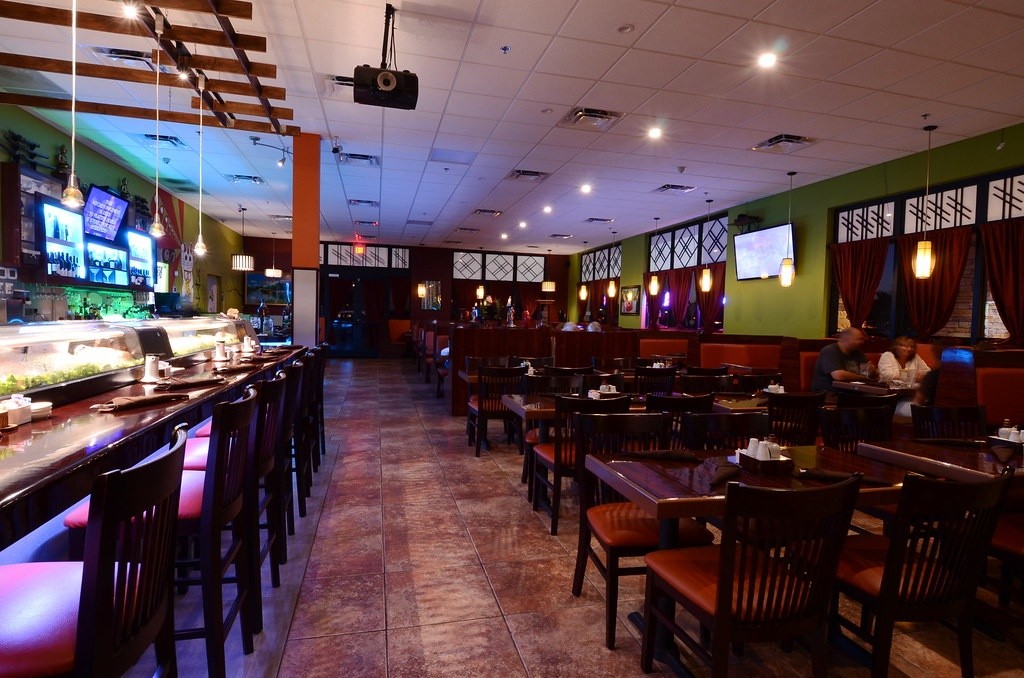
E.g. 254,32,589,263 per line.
135,216,152,232
100,295,133,316
768,379,779,392
47,252,80,278
282,302,293,333
256,299,270,333
88,250,122,269
998,419,1017,439
130,266,151,285
768,434,780,458
133,292,148,306
600,381,609,392
125,306,152,319
66,294,101,320
598,309,605,324
47,213,69,241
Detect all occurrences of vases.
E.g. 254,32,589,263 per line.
506,305,516,327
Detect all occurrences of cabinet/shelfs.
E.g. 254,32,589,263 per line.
0,160,156,295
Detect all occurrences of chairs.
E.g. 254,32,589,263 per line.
0,323,1024,678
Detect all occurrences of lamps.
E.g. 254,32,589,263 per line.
147,14,166,238
476,247,484,299
909,124,940,281
192,74,208,256
778,170,798,288
265,232,282,278
578,241,589,300
59,0,86,209
541,250,556,292
648,217,661,296
231,207,255,272
417,243,426,298
607,231,618,298
698,199,714,292
278,150,286,167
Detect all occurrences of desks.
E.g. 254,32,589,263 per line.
457,367,604,449
680,391,779,449
646,353,687,367
583,443,937,678
830,376,920,442
593,366,681,393
501,391,648,520
720,361,780,391
857,431,1024,659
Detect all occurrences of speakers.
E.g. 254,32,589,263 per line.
353,66,419,110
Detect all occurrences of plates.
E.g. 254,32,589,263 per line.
31,402,53,419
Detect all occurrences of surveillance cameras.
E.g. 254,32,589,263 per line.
332,145,342,153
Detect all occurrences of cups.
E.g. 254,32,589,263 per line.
609,385,616,392
272,316,282,332
528,367,534,376
747,438,770,460
0,406,8,428
250,317,261,334
263,319,274,336
779,386,784,393
1009,430,1024,441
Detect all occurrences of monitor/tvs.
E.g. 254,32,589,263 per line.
733,221,796,281
33,183,158,292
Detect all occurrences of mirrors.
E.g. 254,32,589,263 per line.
420,279,442,310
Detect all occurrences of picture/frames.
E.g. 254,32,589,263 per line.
619,284,641,316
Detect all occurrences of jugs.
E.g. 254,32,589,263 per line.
243,337,251,351
145,354,160,381
216,340,225,359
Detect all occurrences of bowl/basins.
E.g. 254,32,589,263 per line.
900,369,919,387
564,322,577,330
588,322,601,332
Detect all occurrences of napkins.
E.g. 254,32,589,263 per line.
153,370,224,392
89,392,190,414
211,362,263,375
264,348,291,354
237,355,279,364
275,344,303,350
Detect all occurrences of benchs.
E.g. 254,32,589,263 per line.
779,334,890,393
632,331,699,359
386,320,412,357
686,333,786,373
975,349,1024,428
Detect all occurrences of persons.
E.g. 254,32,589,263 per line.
812,327,873,406
878,336,931,417
